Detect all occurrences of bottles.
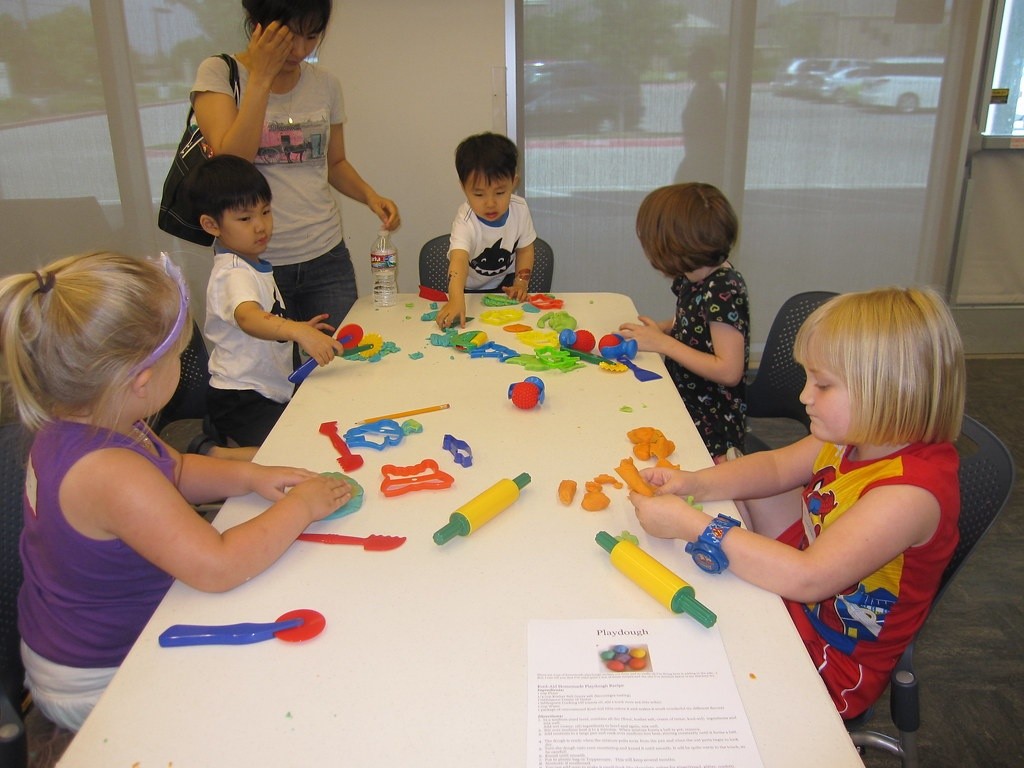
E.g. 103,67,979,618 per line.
369,228,399,307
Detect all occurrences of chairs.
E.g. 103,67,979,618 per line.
418,235,555,293
0,421,225,768
744,292,842,457
144,313,237,455
850,415,1016,768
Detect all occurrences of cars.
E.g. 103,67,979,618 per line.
773,54,868,106
520,57,645,140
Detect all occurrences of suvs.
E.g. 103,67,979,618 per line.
856,55,947,115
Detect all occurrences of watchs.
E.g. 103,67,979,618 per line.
683,513,741,575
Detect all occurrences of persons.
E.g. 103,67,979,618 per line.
0,250,351,734
189,0,400,338
437,133,538,328
612,182,751,460
190,155,344,462
629,288,967,719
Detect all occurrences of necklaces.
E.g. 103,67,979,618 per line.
269,70,293,124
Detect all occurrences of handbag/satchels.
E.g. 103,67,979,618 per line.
158,53,241,247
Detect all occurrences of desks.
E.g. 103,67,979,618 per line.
53,293,869,768
